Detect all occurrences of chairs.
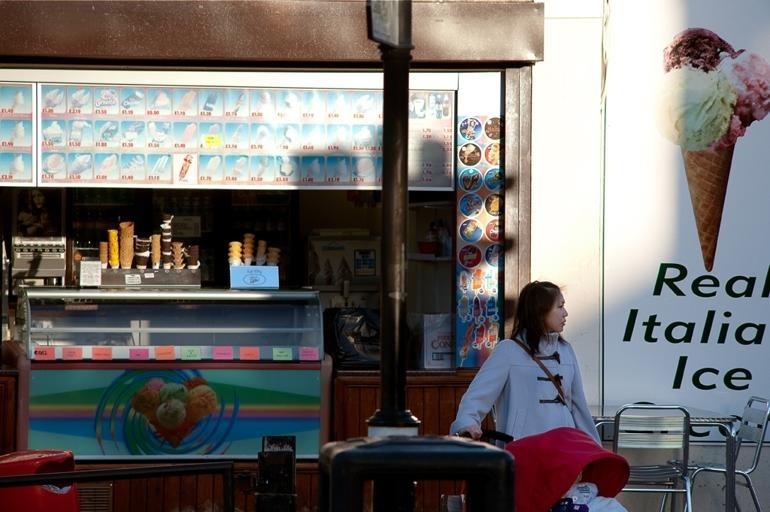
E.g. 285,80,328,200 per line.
596,393,770,512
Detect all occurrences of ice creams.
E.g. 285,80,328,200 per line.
133,376,216,447
653,28,770,271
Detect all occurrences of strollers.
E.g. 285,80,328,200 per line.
457,427,629,512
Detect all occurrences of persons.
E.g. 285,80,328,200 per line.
449,280,599,451
18,189,49,237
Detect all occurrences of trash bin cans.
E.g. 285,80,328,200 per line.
0,447,80,512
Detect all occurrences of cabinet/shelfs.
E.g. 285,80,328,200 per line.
407,199,453,263
18,287,325,363
